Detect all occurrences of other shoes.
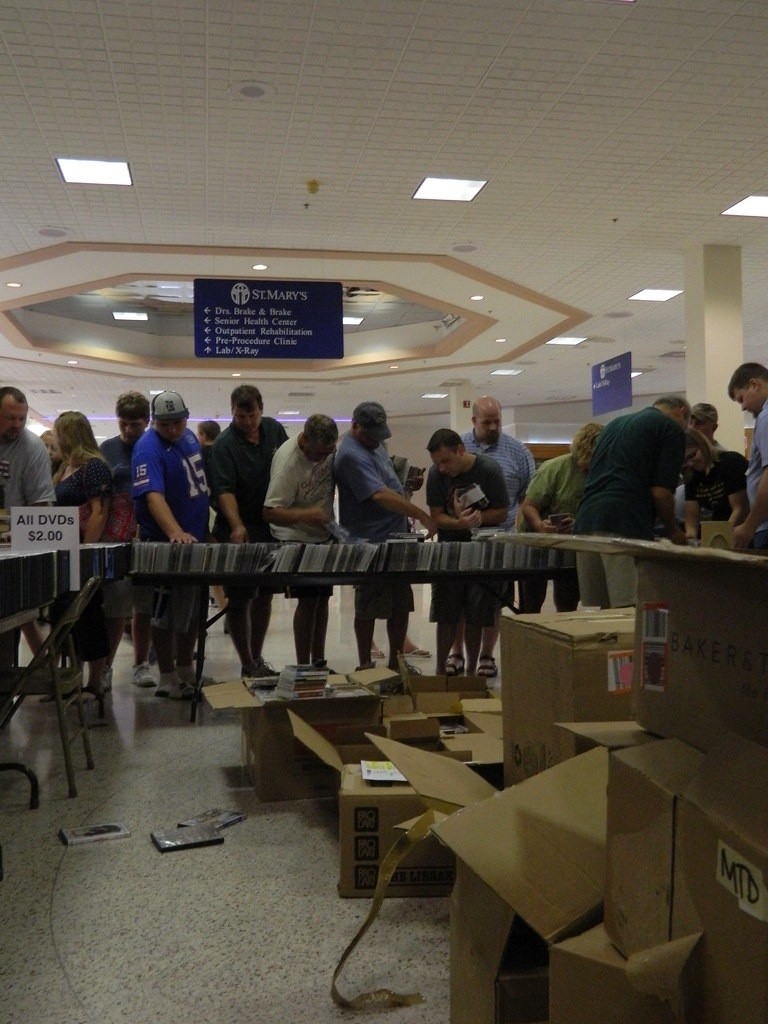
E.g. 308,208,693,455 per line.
241,657,282,690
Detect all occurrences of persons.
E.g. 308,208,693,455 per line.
261,413,340,676
94,389,158,689
1,386,59,705
446,396,536,677
129,389,209,702
331,401,438,677
39,410,115,705
679,426,753,547
515,423,605,615
727,362,767,549
571,392,692,609
425,428,511,677
685,402,727,451
195,384,292,682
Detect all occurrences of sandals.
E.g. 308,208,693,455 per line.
476,655,498,678
445,654,465,676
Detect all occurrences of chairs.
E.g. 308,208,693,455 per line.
0,574,108,805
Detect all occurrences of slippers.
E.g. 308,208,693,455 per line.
70,680,105,705
370,648,385,658
155,675,217,699
39,687,84,702
401,648,432,657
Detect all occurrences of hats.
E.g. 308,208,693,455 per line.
151,391,189,421
353,400,391,442
691,402,718,424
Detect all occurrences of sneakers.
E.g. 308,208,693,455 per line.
131,664,155,686
103,667,113,691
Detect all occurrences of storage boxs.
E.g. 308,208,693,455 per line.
195,535,768,1024
